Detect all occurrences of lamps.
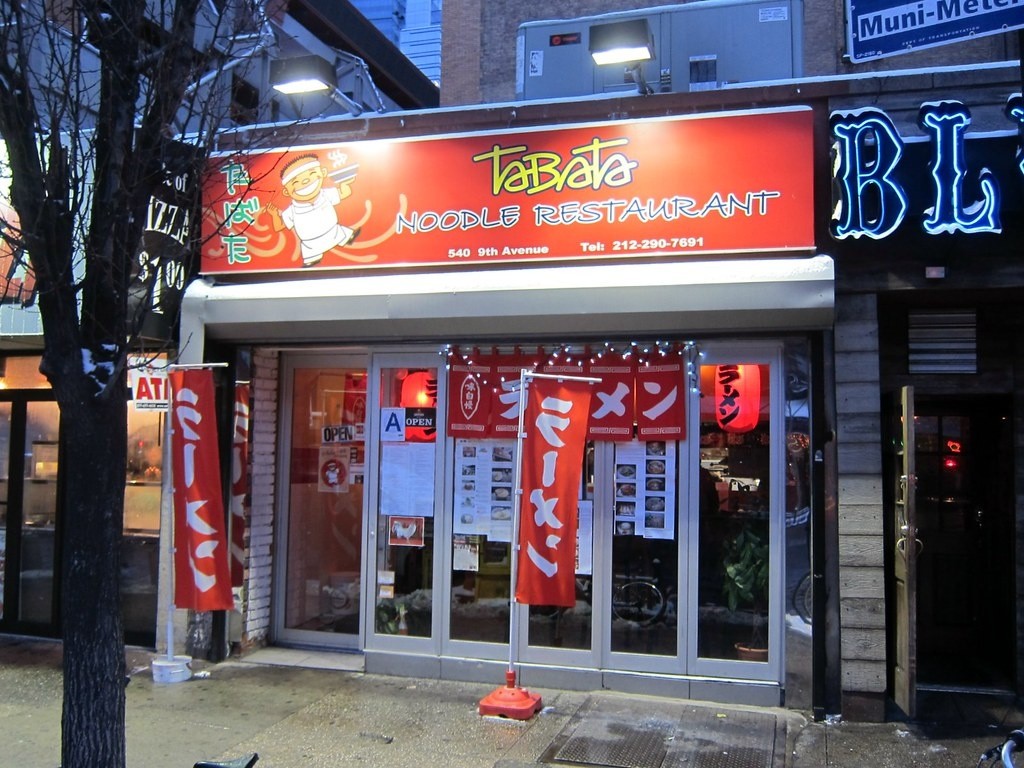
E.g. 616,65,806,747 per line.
269,54,363,117
589,17,657,96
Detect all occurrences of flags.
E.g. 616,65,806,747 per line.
167,368,234,611
515,379,591,606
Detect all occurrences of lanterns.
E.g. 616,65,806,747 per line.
399,372,436,439
715,364,760,433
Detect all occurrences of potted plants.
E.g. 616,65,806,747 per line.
720,524,769,662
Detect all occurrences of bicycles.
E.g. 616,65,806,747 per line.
546,567,666,626
